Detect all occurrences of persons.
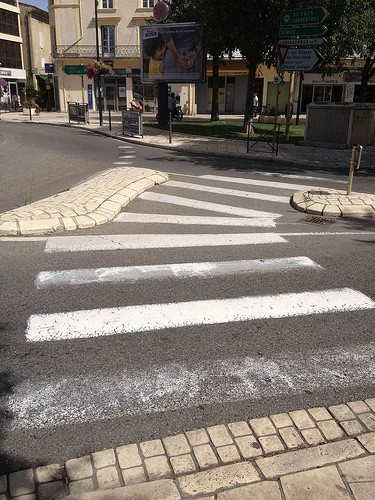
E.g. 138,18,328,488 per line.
252,93,259,117
135,101,140,109
159,27,200,78
144,32,167,79
168,91,177,123
131,98,136,109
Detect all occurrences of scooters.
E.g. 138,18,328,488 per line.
126,102,143,114
252,104,279,117
156,105,183,121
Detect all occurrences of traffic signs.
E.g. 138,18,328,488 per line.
276,37,329,48
279,5,331,27
276,24,328,39
277,48,324,72
